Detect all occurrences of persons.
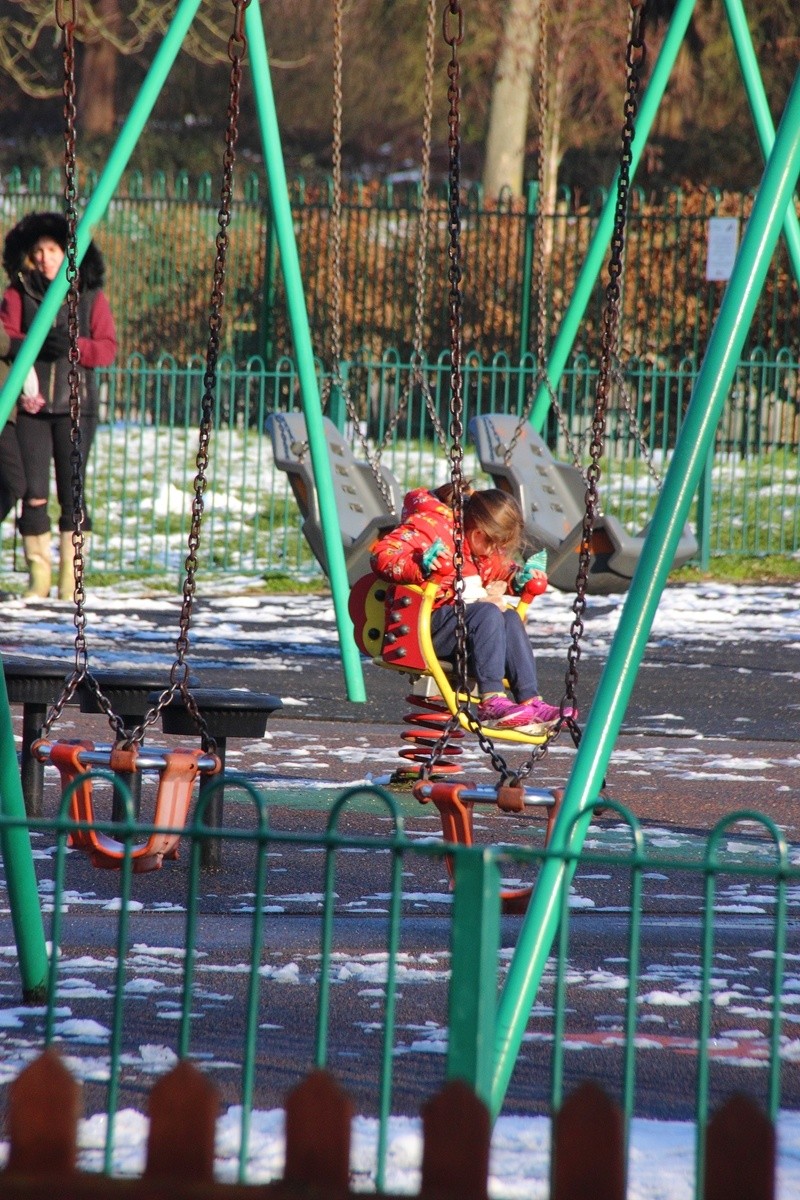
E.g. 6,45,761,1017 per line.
370,479,578,736
0,212,117,599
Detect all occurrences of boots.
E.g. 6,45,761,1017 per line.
57,530,83,601
21,532,51,598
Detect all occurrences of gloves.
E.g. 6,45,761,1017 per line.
43,326,72,354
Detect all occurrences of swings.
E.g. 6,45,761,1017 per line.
412,1,647,902
30,1,252,876
474,4,699,598
268,1,474,589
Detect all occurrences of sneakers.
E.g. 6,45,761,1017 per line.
517,695,578,732
477,695,535,730
